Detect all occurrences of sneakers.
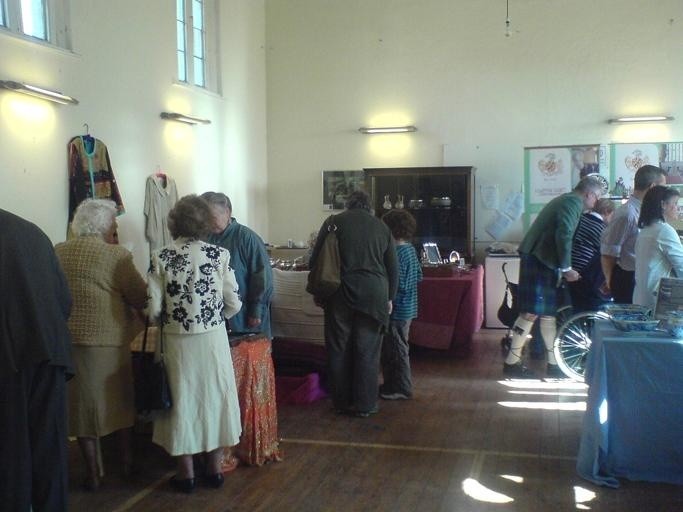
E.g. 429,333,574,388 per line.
378,383,416,399
334,403,378,418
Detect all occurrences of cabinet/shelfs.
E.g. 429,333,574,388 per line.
361,164,479,261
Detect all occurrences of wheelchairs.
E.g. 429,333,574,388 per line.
551,275,614,383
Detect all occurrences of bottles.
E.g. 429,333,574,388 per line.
267,257,307,271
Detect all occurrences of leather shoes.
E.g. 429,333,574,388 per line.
83,463,101,486
208,473,225,490
177,478,198,495
503,361,539,379
545,362,570,378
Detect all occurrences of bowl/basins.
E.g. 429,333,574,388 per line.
599,303,660,334
406,199,424,210
663,305,683,337
429,197,451,210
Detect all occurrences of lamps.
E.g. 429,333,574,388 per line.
0,79,80,108
357,125,418,137
159,110,211,128
607,115,675,126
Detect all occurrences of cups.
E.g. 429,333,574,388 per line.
650,278,682,332
287,239,292,248
264,244,273,250
440,258,464,270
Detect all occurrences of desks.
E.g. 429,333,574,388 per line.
573,316,682,489
268,245,519,354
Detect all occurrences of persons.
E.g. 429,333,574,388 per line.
0,209,73,512
568,198,614,341
55,197,149,492
305,188,400,419
198,192,283,472
631,186,683,314
598,165,667,303
379,210,423,400
141,191,244,494
502,172,610,385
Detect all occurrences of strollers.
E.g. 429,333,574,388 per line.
500,260,546,360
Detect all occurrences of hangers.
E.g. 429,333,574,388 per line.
79,123,94,145
153,164,167,180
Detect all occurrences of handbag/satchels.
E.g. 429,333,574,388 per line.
132,360,171,410
306,231,341,299
